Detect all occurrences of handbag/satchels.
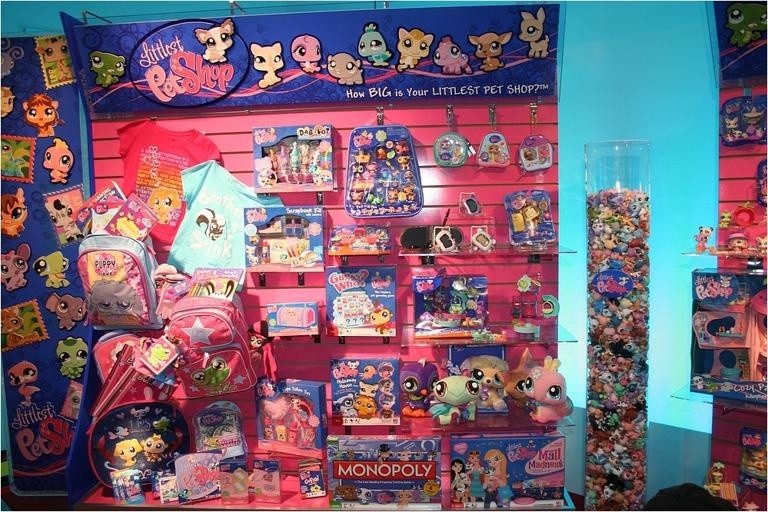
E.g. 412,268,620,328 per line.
88,338,179,416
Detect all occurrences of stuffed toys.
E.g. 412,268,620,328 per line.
400,357,574,424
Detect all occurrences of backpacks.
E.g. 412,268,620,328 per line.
88,400,190,489
77,233,165,332
169,296,257,399
